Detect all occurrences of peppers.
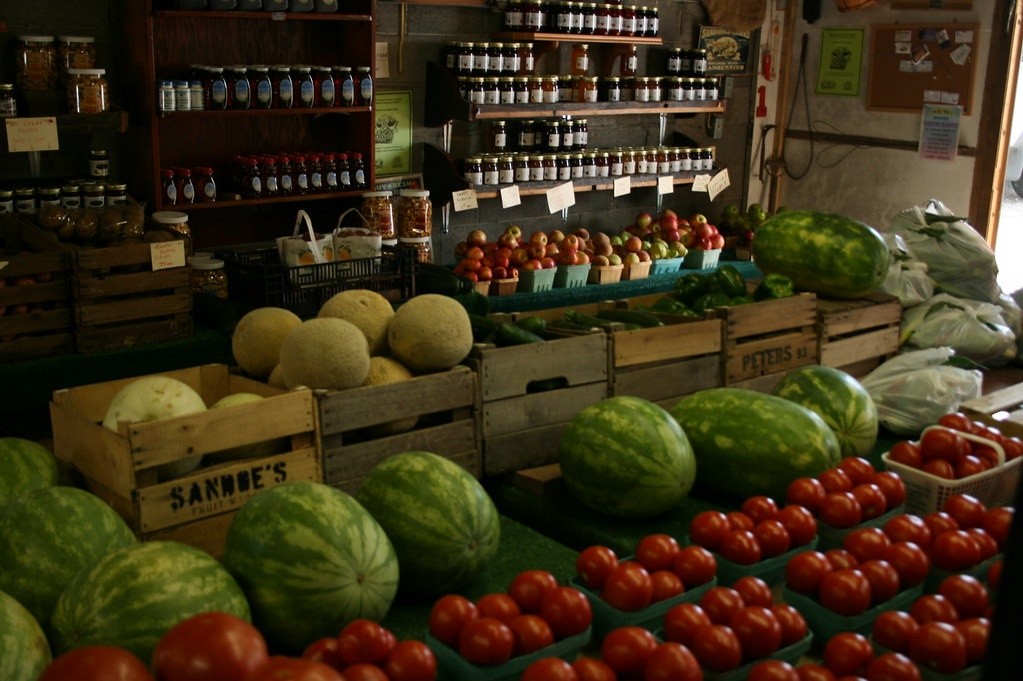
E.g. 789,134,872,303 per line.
636,264,795,314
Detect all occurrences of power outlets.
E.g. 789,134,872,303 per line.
707,117,723,139
721,76,734,98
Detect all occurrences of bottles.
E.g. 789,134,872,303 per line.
160,64,373,111
228,151,366,199
161,165,216,204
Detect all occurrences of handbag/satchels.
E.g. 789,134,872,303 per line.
862,345,983,439
877,196,1023,367
278,207,383,288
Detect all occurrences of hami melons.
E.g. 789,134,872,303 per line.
230,289,473,438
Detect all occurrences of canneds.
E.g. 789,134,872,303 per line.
444,1,719,105
462,117,715,185
361,189,433,265
0,148,127,215
0,36,113,118
151,211,228,305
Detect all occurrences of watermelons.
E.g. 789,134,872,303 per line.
357,450,499,583
770,365,876,459
47,539,253,663
752,210,890,299
0,436,144,681
222,480,400,645
559,394,696,517
665,387,842,508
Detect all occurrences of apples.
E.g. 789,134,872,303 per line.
450,204,767,285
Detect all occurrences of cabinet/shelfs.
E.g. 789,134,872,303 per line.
0,0,723,221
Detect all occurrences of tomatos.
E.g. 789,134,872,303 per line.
37,412,1023,681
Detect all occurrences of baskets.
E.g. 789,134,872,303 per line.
881,423,1023,513
473,248,723,300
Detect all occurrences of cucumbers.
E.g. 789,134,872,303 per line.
467,312,551,349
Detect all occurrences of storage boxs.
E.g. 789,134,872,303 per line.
308,365,483,499
49,364,325,560
615,282,819,395
16,211,193,355
491,276,519,295
213,245,406,318
459,312,609,473
504,298,724,404
0,213,74,362
473,281,490,297
553,263,590,289
517,267,557,295
682,248,722,269
424,378,1023,681
620,260,652,281
650,257,685,276
589,264,625,285
744,279,904,380
